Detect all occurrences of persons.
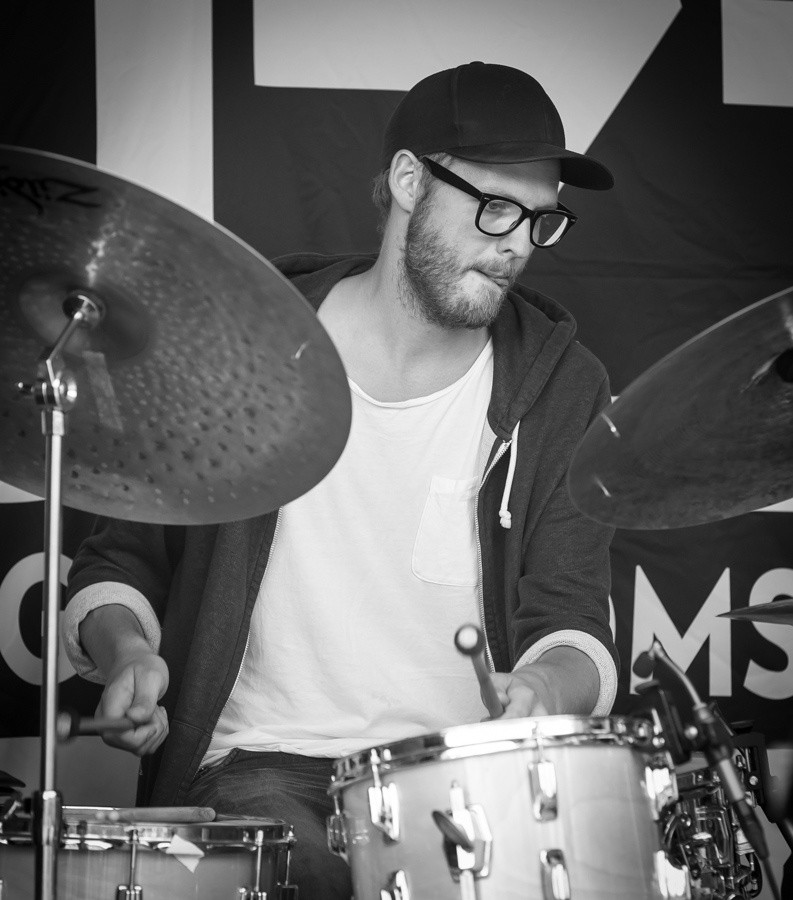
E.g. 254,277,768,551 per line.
60,60,622,900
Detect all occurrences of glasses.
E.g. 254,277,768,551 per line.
426,157,578,250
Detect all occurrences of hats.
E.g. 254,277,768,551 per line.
388,62,614,191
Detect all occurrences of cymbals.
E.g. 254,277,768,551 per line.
713,597,793,625
2,144,354,530
567,286,793,533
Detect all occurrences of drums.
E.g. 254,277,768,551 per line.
331,715,669,900
2,805,294,900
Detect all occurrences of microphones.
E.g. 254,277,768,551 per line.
632,641,769,862
